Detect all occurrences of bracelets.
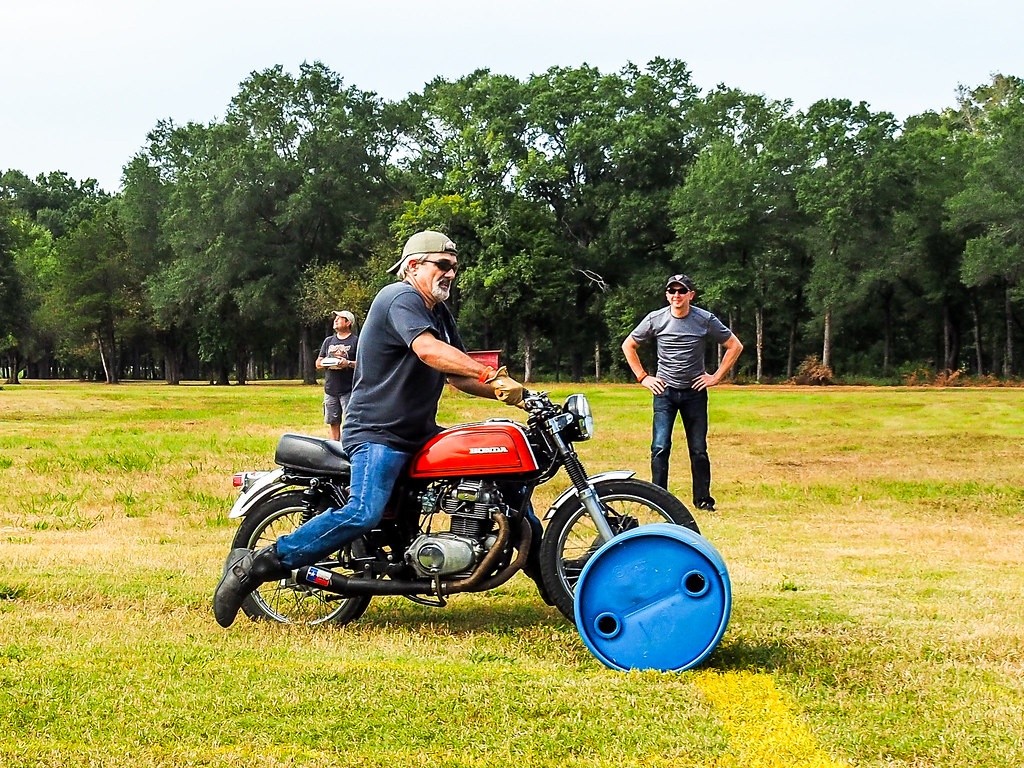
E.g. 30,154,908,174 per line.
480,366,495,384
637,372,648,383
348,361,352,368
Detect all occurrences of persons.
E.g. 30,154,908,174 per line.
620,274,744,513
213,231,526,628
316,310,359,441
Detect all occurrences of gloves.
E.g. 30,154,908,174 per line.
485,366,526,410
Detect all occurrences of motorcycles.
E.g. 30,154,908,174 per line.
227,388,701,628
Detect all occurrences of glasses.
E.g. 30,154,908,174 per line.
667,288,690,294
423,259,458,274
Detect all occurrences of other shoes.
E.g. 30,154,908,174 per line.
699,503,715,511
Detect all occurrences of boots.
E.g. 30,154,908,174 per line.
213,545,292,628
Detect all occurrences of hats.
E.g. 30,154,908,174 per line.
333,311,355,327
385,231,459,273
667,274,693,293
